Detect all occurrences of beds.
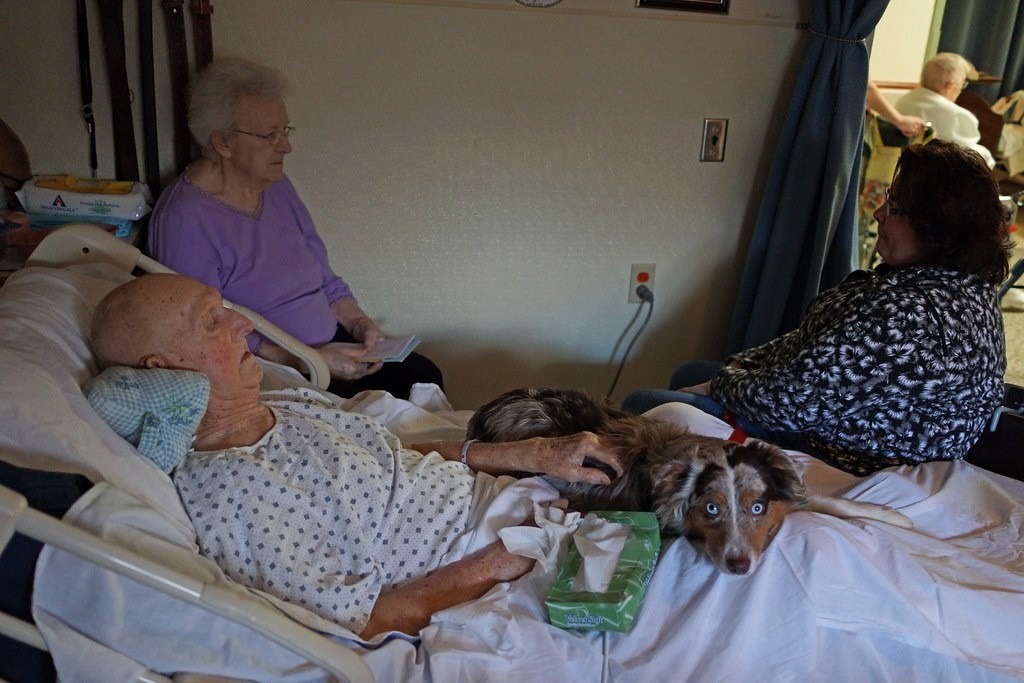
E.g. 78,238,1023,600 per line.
0,219,1024,683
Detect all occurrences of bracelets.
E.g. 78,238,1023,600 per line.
461,439,482,464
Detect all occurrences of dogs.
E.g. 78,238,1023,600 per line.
466,386,912,578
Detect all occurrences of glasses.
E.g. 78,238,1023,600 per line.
231,126,296,145
884,187,905,216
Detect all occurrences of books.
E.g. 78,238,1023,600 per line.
354,333,421,361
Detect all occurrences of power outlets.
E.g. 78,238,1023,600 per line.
628,260,656,304
698,117,732,166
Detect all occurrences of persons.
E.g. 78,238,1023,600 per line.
89,274,629,641
890,50,996,170
148,57,444,401
622,139,1016,477
864,80,925,139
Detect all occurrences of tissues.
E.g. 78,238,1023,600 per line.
543,510,662,634
